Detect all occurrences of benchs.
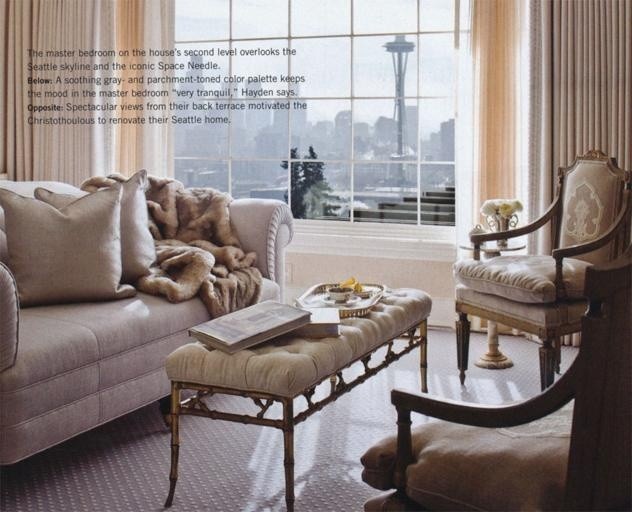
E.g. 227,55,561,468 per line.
164,286,433,510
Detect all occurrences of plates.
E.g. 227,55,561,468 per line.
319,293,362,308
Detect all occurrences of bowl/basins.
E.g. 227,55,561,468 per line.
326,286,355,303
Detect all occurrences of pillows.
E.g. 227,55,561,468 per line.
0,168,159,309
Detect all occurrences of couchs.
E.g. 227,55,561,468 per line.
0,178,294,470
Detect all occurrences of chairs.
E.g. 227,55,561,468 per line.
360,148,632,511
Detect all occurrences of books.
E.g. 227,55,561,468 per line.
288,307,341,339
188,300,312,355
